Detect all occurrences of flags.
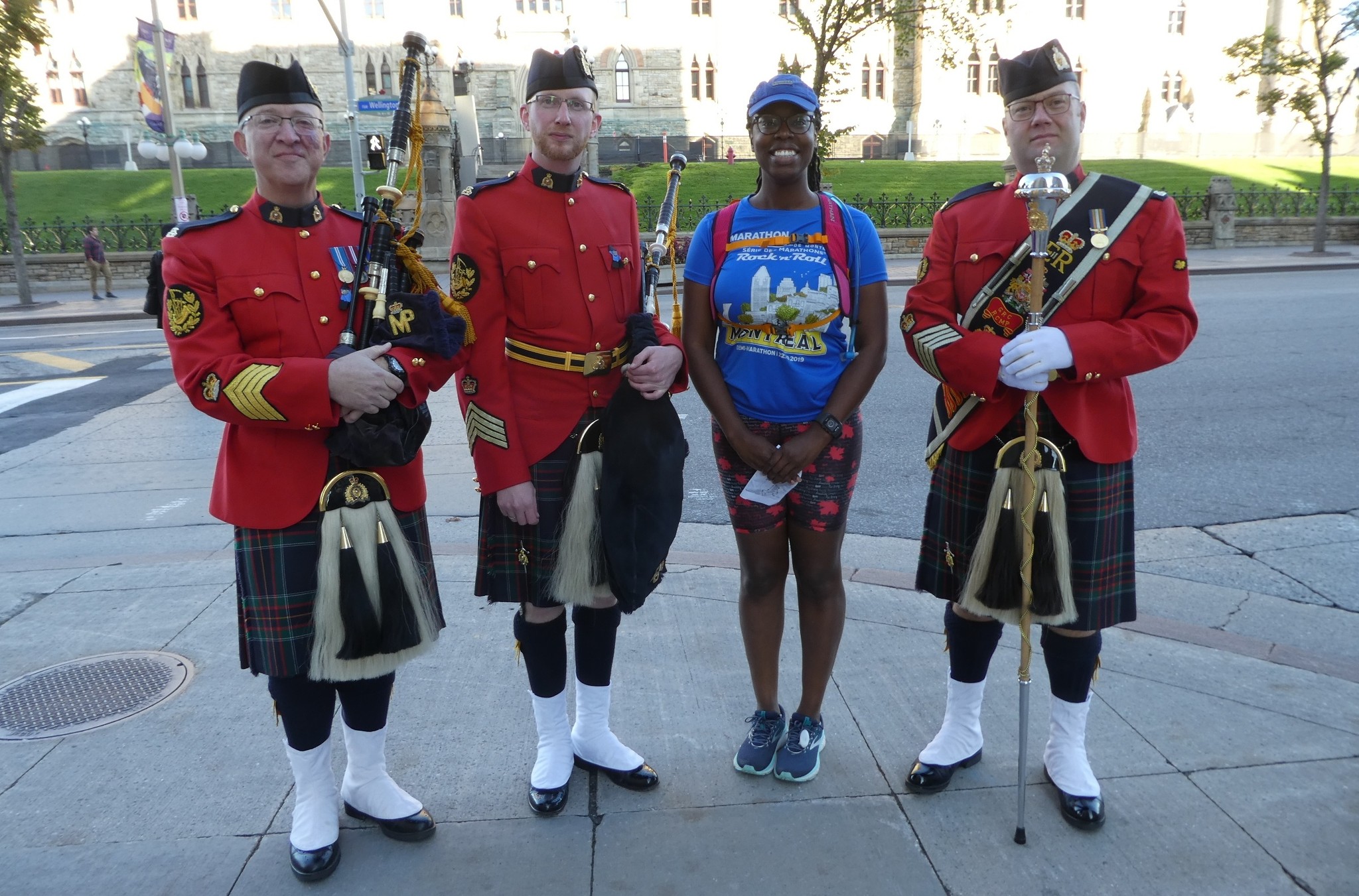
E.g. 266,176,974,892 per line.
132,19,174,135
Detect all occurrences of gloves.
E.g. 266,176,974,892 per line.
998,357,1050,392
998,325,1073,380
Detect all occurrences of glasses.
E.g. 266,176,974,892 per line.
751,112,820,134
237,112,325,135
1006,91,1081,122
526,93,594,116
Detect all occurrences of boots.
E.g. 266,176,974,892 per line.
513,601,575,816
268,673,340,883
336,669,437,842
1040,623,1105,831
904,599,1006,793
570,601,660,792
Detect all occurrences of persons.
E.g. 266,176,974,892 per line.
899,41,1202,830
83,226,118,301
159,58,472,881
450,47,691,818
150,224,176,329
683,72,889,783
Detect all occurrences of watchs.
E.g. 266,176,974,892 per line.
381,353,407,385
814,412,843,439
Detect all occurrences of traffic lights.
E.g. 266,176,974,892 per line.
367,134,387,171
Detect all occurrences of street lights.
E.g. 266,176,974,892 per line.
74,116,94,168
138,0,208,223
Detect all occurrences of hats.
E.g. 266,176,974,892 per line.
746,73,821,118
525,44,599,103
997,38,1079,107
237,59,324,128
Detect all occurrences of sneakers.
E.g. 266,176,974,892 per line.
106,293,118,298
733,701,789,775
773,712,827,782
92,296,104,300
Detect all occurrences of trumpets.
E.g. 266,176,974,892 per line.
629,151,688,347
306,28,479,359
